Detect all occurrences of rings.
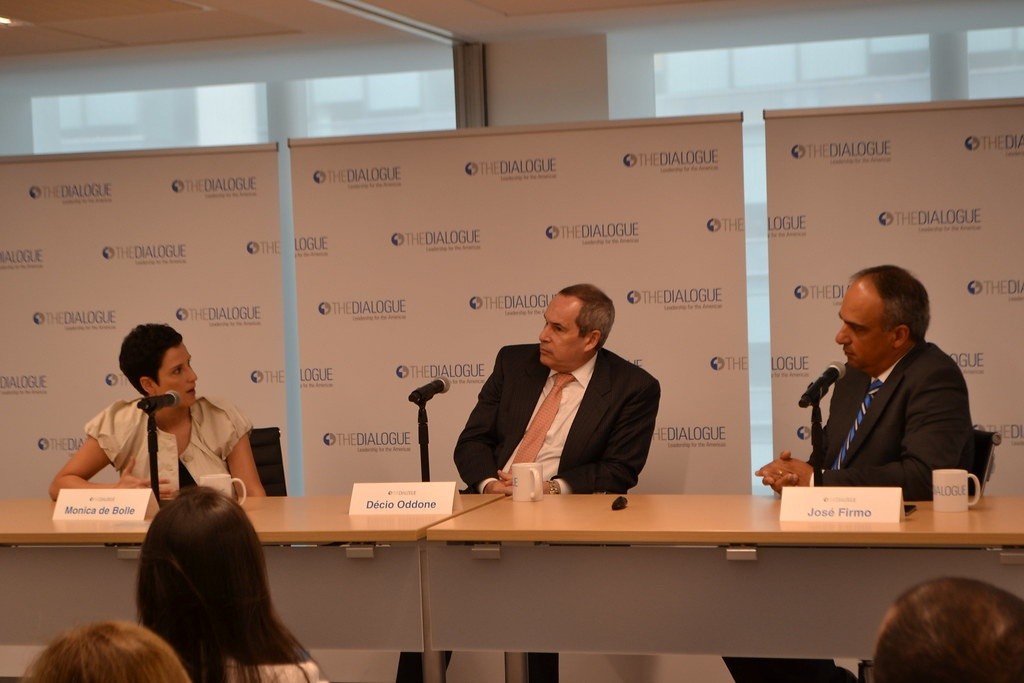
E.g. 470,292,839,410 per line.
779,470,783,476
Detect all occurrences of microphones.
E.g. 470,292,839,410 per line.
799,360,846,408
408,376,451,402
137,390,181,411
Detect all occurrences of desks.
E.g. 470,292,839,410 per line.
0,492,1024,683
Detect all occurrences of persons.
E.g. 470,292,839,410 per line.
49,324,267,501
722,265,975,683
872,578,1024,683
138,487,329,683
395,283,662,683
19,620,192,683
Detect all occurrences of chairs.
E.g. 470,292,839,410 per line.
859,427,1002,682
247,427,287,497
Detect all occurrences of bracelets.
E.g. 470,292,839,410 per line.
546,480,559,494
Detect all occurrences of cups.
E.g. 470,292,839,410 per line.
199,474,246,506
512,463,543,501
932,469,980,512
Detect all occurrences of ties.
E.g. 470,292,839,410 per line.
508,372,575,477
833,379,883,472
